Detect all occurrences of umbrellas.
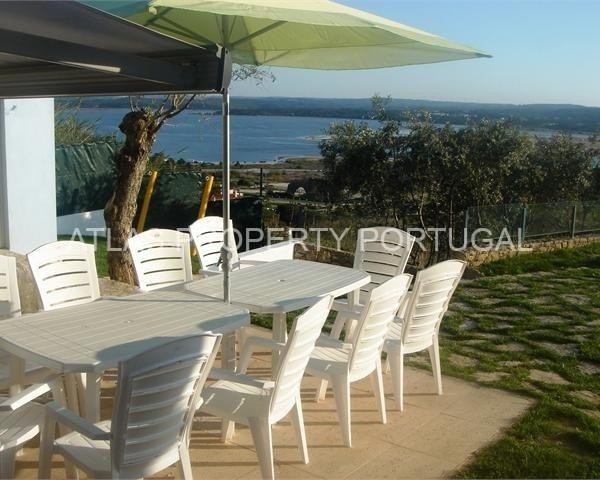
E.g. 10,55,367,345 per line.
76,0,494,302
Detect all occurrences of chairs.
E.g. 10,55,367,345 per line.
0,216,469,480
